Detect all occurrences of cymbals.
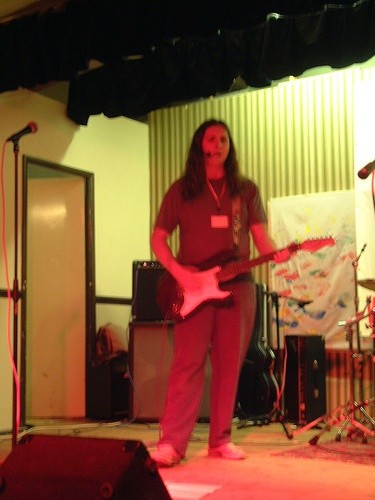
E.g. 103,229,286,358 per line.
355,278,375,292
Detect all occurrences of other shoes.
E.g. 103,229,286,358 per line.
150,442,180,466
207,440,247,460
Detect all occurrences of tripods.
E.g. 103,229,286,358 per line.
238,258,375,445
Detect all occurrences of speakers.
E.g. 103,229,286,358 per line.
0,432,171,500
129,321,212,421
283,334,327,421
86,353,131,419
132,260,168,320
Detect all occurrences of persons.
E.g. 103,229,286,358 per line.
148,119,290,465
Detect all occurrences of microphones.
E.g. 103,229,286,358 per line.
358,159,375,179
203,153,211,158
6,121,37,141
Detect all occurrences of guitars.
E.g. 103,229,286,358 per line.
152,234,335,323
